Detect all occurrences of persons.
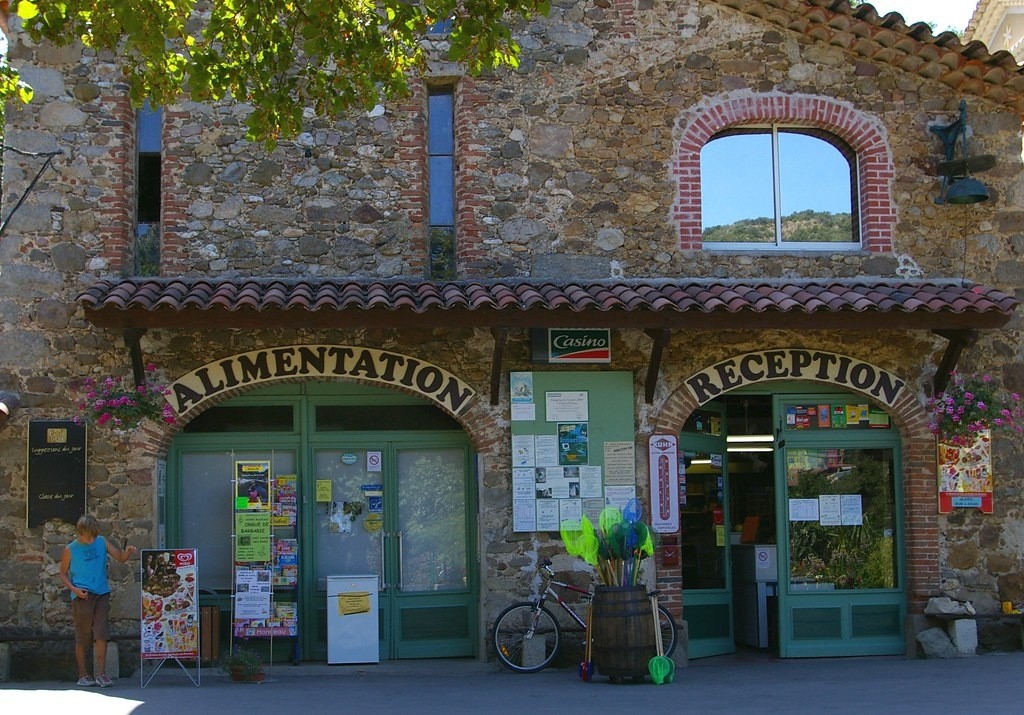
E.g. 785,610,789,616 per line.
59,515,139,688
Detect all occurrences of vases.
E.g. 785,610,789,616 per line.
230,666,248,681
115,409,144,424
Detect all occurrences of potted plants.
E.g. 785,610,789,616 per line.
255,665,265,680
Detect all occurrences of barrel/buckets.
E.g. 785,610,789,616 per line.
591,583,657,678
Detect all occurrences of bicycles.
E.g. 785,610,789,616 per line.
491,559,676,673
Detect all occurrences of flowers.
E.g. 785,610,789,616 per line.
72,362,174,427
926,371,1024,436
217,644,260,677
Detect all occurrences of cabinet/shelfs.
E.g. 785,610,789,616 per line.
730,545,778,648
681,473,719,576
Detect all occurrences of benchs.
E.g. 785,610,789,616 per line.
491,623,684,667
937,612,1024,656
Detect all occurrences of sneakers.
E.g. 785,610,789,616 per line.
96,675,114,688
77,675,96,686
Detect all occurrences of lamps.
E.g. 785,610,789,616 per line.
691,400,775,474
929,99,997,205
0,392,21,433
